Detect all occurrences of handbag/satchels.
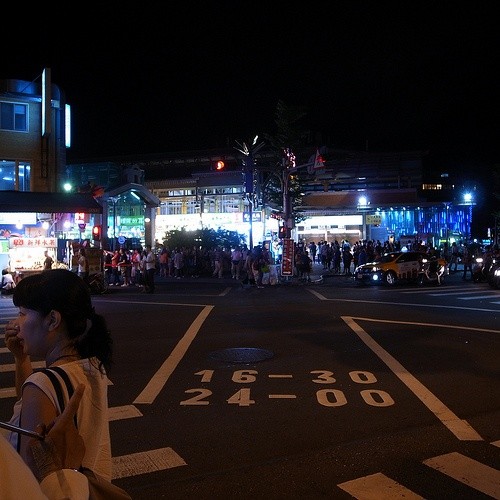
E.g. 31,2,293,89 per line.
16,368,133,500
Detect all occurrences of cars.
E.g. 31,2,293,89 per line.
353,252,449,286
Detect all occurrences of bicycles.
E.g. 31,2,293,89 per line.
80,273,109,294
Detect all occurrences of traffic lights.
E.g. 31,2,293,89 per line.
204,160,232,171
92,226,102,241
279,225,285,238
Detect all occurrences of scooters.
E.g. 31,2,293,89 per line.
415,255,445,287
470,250,500,289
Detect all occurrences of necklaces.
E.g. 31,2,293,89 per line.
48,354,80,366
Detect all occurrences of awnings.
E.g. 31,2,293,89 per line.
0,189,104,214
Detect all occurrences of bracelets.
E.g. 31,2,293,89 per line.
72,468,78,472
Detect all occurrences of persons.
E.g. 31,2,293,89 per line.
0,239,91,295
293,235,500,290
103,239,283,294
0,383,90,500
5,270,114,485
